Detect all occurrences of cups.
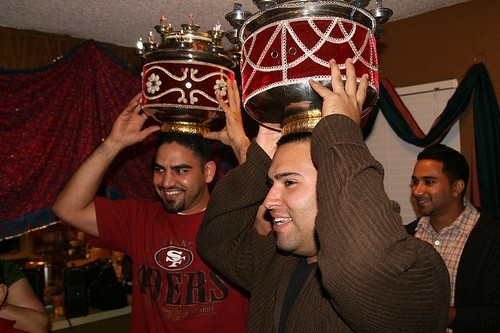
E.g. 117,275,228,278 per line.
63,267,90,319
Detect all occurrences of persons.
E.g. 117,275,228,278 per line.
53,78,252,333
0,259,49,333
403,141,500,333
196,58,450,333
